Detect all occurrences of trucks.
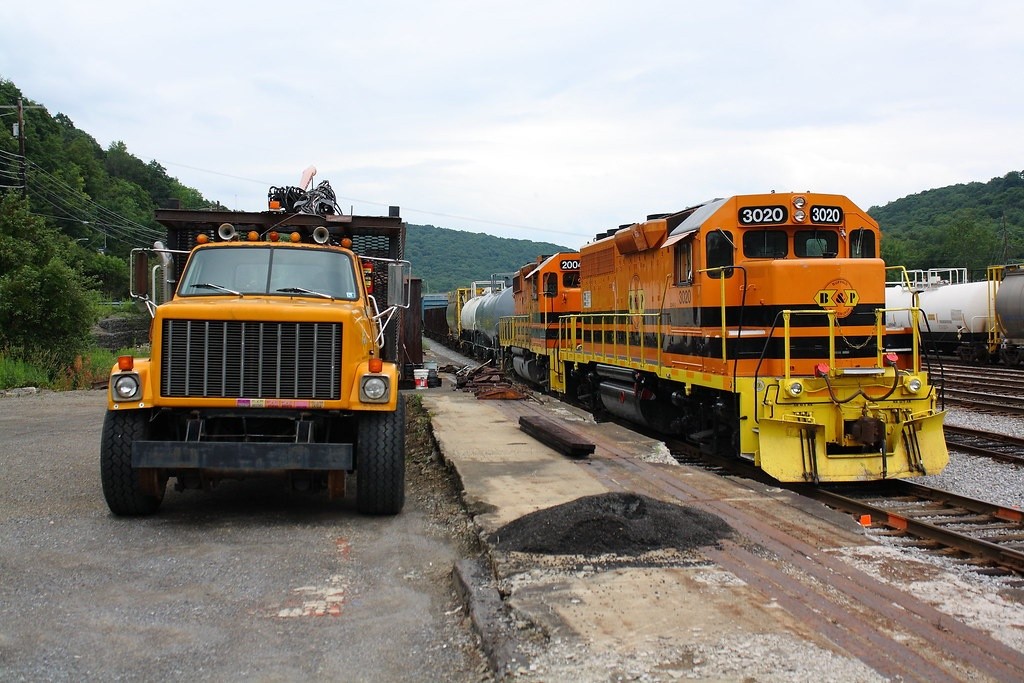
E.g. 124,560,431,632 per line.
96,203,424,517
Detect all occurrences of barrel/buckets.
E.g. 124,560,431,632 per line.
405,363,423,379
427,369,438,387
414,369,428,389
423,360,439,376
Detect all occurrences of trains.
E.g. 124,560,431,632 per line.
884,265,1024,372
446,189,949,484
